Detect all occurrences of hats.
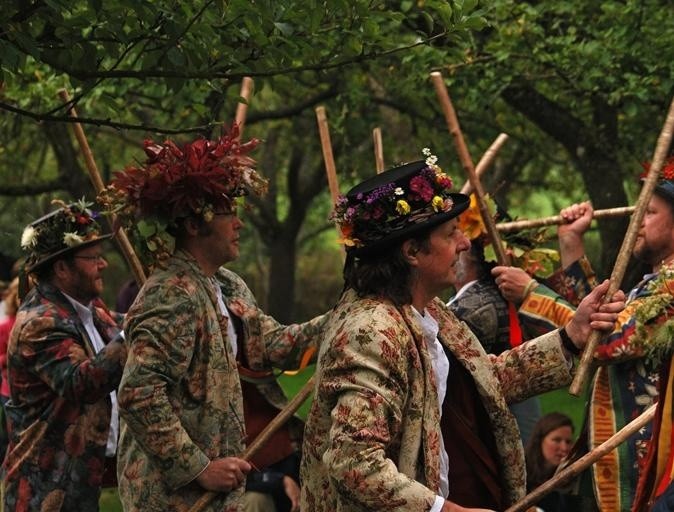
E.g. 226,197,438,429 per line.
26,203,112,275
346,160,471,253
638,174,674,206
